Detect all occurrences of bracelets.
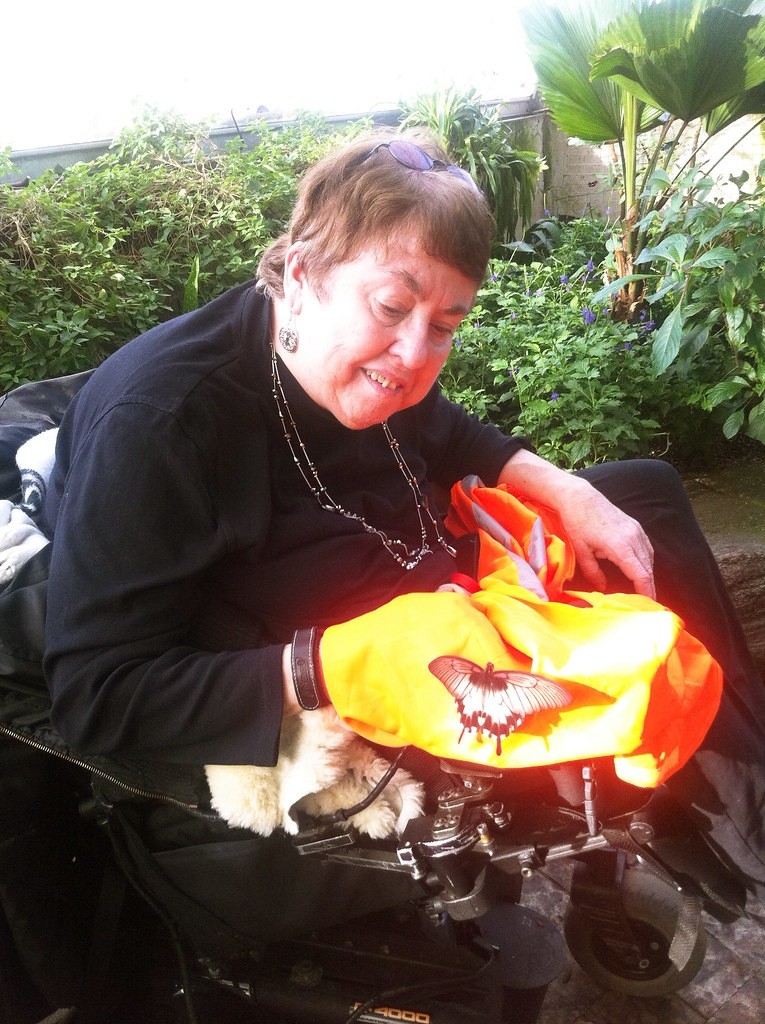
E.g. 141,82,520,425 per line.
289,625,333,711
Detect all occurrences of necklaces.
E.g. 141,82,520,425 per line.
266,334,459,570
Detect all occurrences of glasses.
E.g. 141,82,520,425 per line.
362,140,472,187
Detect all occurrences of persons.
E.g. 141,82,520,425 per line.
39,131,764,892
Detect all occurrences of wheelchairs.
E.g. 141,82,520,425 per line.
2,370,763,1024
